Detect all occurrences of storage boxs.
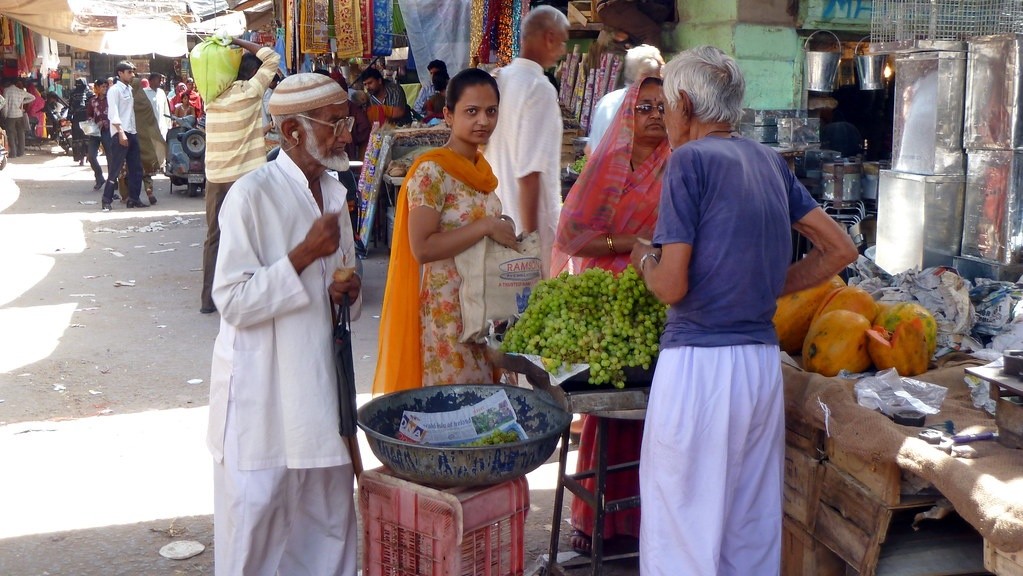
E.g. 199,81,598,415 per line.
359,466,532,576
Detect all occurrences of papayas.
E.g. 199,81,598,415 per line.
774,274,937,377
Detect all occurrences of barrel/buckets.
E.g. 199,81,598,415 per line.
803,29,842,92
853,35,889,90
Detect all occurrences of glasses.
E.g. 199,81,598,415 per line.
635,103,664,115
296,114,355,136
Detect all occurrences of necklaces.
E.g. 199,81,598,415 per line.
706,128,733,138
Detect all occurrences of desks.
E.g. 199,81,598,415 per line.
545,408,647,576
383,173,405,218
341,160,381,249
761,141,822,175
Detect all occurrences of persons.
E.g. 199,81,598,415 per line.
482,5,576,287
211,67,368,576
0,60,461,319
371,69,524,396
198,31,282,313
636,43,857,575
583,43,663,166
549,74,687,553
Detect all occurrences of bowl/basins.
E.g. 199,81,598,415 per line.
356,384,574,487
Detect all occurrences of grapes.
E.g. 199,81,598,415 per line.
572,155,586,173
460,429,518,447
501,263,672,387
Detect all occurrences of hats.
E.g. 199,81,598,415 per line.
268,72,348,115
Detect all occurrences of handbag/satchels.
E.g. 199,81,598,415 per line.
79,119,100,137
454,214,546,344
333,292,357,436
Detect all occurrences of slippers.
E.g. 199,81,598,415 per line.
570,530,591,557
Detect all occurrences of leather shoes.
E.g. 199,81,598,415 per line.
126,199,149,208
102,202,112,210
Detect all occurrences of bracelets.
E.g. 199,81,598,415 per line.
639,254,659,272
607,232,617,255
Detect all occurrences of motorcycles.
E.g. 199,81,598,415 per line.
42,92,72,155
162,114,206,198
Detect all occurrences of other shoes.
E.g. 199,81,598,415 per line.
94,180,105,190
113,194,120,200
147,190,157,205
114,181,118,190
